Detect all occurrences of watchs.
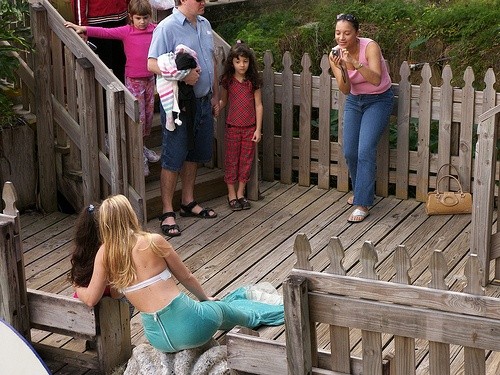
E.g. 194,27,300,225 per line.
355,63,364,71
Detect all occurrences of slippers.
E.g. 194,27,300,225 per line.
347,195,355,205
347,208,371,223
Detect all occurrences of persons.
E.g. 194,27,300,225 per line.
329,14,395,223
215,40,264,211
72,194,284,353
68,202,136,351
147,0,219,237
70,0,131,155
64,0,160,176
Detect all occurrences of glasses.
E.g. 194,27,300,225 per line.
336,14,355,21
195,0,203,3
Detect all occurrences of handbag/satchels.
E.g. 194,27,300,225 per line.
426,164,473,216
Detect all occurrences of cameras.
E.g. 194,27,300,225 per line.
332,48,342,61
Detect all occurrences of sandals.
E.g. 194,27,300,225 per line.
228,194,251,210
180,200,218,218
159,211,182,236
